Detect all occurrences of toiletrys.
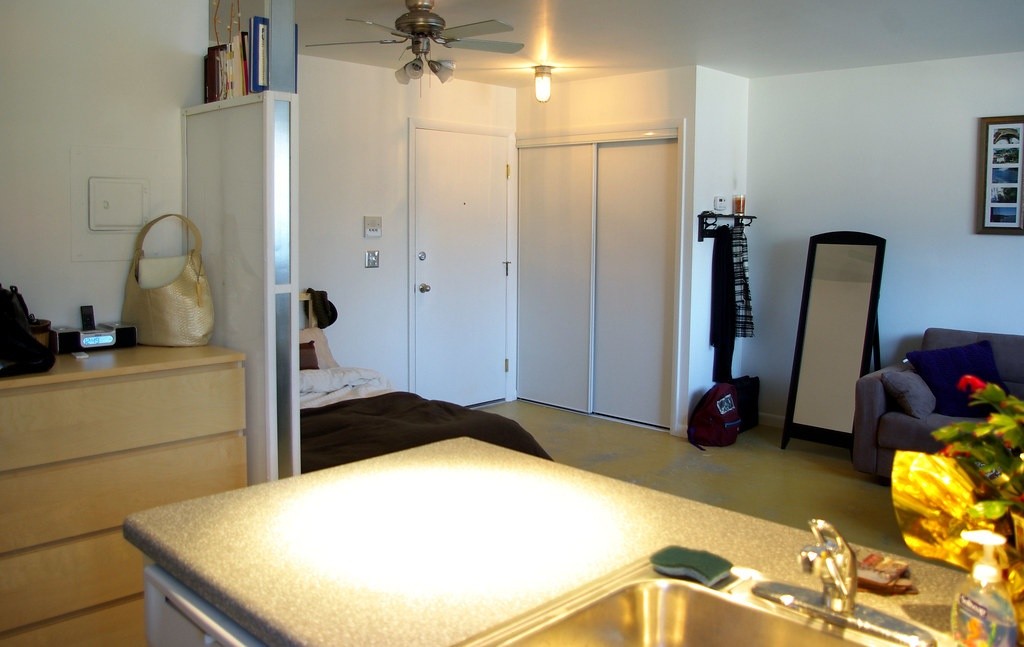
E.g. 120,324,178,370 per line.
950,528,1019,647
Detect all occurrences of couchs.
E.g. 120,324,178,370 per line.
854,328,1024,485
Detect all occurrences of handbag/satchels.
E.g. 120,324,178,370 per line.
121,213,215,347
732,373,760,434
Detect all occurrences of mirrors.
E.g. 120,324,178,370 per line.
781,231,886,448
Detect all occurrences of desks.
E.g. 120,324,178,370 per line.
0,343,249,647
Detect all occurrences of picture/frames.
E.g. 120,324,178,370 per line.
976,115,1024,235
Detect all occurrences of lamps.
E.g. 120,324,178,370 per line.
394,37,455,85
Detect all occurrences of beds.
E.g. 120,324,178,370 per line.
300,292,554,483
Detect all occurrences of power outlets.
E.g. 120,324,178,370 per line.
365,251,379,268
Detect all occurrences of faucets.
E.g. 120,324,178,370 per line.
794,517,858,617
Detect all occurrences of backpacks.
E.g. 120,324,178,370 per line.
686,383,741,451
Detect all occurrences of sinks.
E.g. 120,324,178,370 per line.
442,549,952,647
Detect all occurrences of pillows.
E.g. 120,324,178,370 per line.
880,368,936,418
908,341,1010,416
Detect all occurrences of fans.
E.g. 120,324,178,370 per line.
305,0,525,53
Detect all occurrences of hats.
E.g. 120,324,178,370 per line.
304,289,337,329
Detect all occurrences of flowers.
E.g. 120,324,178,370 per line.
930,379,1024,515
